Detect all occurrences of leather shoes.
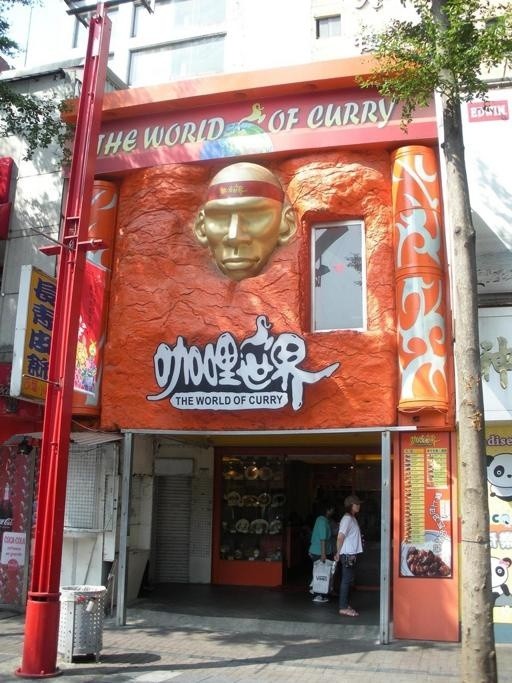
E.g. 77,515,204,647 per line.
338,605,359,616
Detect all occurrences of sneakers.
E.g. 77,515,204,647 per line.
311,596,329,603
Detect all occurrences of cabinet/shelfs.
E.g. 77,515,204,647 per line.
213,448,286,586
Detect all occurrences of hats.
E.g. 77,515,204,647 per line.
344,494,365,506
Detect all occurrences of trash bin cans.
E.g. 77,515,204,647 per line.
56,585,107,664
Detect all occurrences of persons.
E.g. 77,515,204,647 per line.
307,498,335,603
334,495,368,616
193,161,297,280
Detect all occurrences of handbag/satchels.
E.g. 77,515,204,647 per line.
311,559,336,595
